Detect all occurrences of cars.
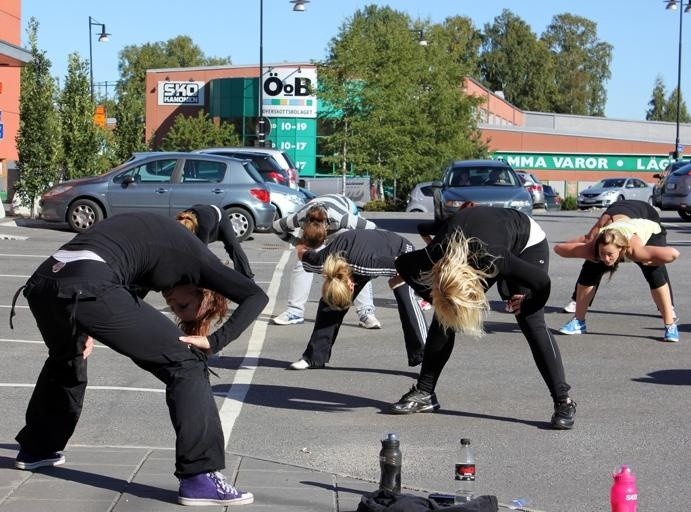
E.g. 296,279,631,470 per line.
652,159,691,206
124,147,318,221
37,150,277,245
577,176,655,210
515,167,562,211
661,162,691,221
405,180,435,214
431,156,535,224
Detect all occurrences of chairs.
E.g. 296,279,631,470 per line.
483,170,500,186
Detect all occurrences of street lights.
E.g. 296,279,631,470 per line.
258,0,314,118
662,0,691,156
89,15,114,106
407,27,428,48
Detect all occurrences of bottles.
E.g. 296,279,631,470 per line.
378,431,404,497
609,461,640,511
453,437,479,505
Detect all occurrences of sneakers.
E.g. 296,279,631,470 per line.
551,397,577,430
14,448,65,470
559,317,587,335
389,383,441,415
289,358,326,370
177,470,255,506
664,318,680,342
272,312,305,326
359,313,382,330
563,302,577,313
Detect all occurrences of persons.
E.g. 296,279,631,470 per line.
15,211,269,506
554,218,681,342
272,193,381,328
391,206,577,430
563,201,680,323
289,229,428,371
417,200,517,314
177,204,255,282
485,171,504,185
456,171,473,186
